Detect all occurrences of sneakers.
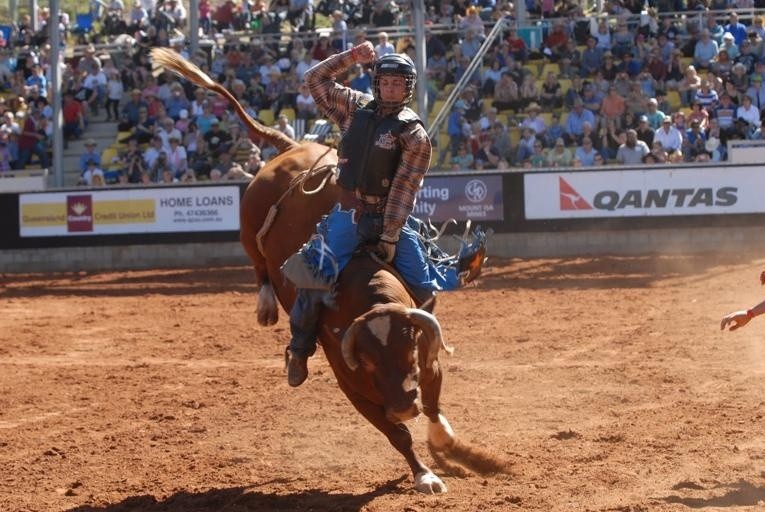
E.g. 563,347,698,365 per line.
287,344,316,387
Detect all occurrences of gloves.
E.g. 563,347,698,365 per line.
352,41,373,63
370,240,396,263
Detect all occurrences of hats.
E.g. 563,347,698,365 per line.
84,0,734,166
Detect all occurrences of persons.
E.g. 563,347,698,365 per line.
275,37,437,389
1,0,765,190
720,270,765,331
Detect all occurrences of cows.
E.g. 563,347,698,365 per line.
149,45,457,496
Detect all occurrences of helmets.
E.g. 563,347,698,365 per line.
371,53,417,76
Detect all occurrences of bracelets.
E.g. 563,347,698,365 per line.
746,310,755,319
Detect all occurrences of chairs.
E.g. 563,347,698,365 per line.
0,0,764,174
